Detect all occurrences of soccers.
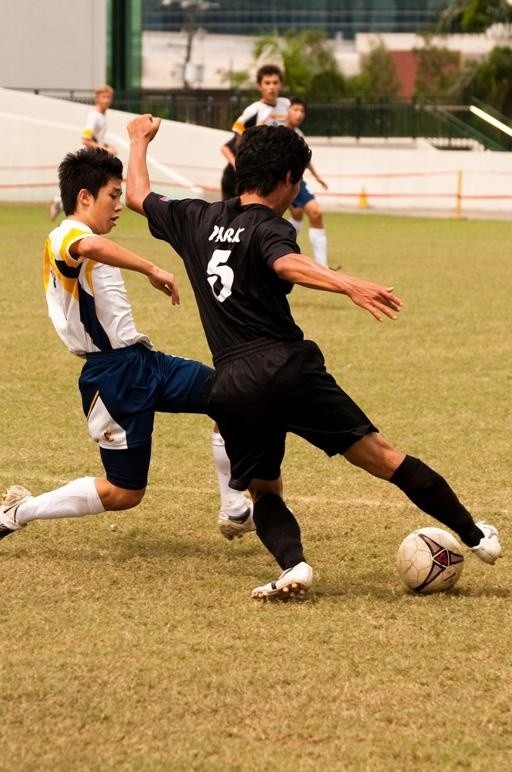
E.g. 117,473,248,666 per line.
395,528,466,594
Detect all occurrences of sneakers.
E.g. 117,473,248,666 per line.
251,560,313,603
218,495,292,539
471,522,504,566
0,484,31,542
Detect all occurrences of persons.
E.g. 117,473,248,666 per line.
222,65,331,271
126,112,504,605
2,146,262,541
48,84,116,222
